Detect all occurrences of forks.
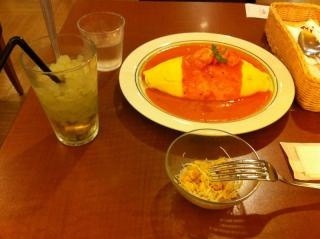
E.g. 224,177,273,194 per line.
209,159,320,190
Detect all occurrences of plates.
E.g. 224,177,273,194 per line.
118,32,296,134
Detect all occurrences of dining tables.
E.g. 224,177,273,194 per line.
2,0,320,238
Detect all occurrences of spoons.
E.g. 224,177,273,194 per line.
298,30,320,69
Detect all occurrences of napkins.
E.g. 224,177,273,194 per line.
245,3,271,19
279,141,320,181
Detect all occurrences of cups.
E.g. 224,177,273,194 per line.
19,33,99,147
77,11,125,72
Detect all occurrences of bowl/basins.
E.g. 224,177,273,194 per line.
164,128,263,210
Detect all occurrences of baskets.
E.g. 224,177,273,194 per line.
265,2,320,112
